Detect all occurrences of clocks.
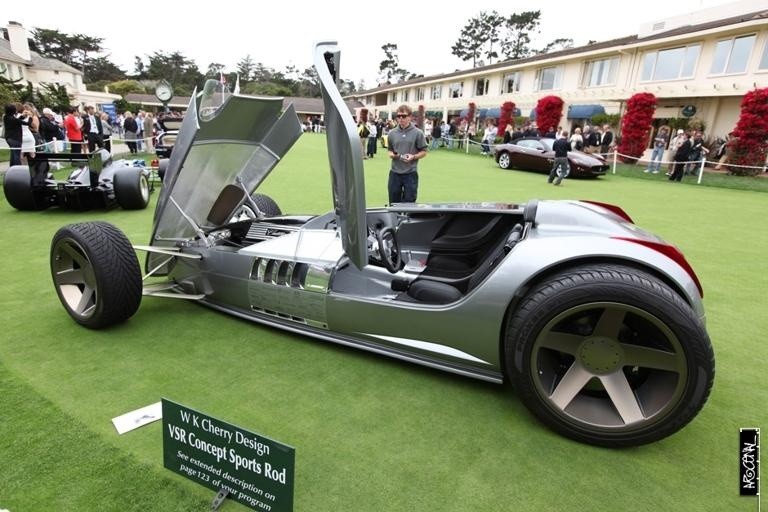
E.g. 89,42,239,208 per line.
155,79,174,104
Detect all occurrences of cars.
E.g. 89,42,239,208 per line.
379,133,430,149
150,114,184,159
3,146,171,212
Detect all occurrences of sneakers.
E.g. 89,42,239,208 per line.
643,169,649,173
652,170,659,174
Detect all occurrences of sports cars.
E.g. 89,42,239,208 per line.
489,135,610,181
42,37,722,450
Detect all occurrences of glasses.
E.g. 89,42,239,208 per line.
396,114,408,118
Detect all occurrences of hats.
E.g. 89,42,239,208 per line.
677,129,683,134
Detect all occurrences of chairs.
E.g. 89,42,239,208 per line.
391,199,539,305
